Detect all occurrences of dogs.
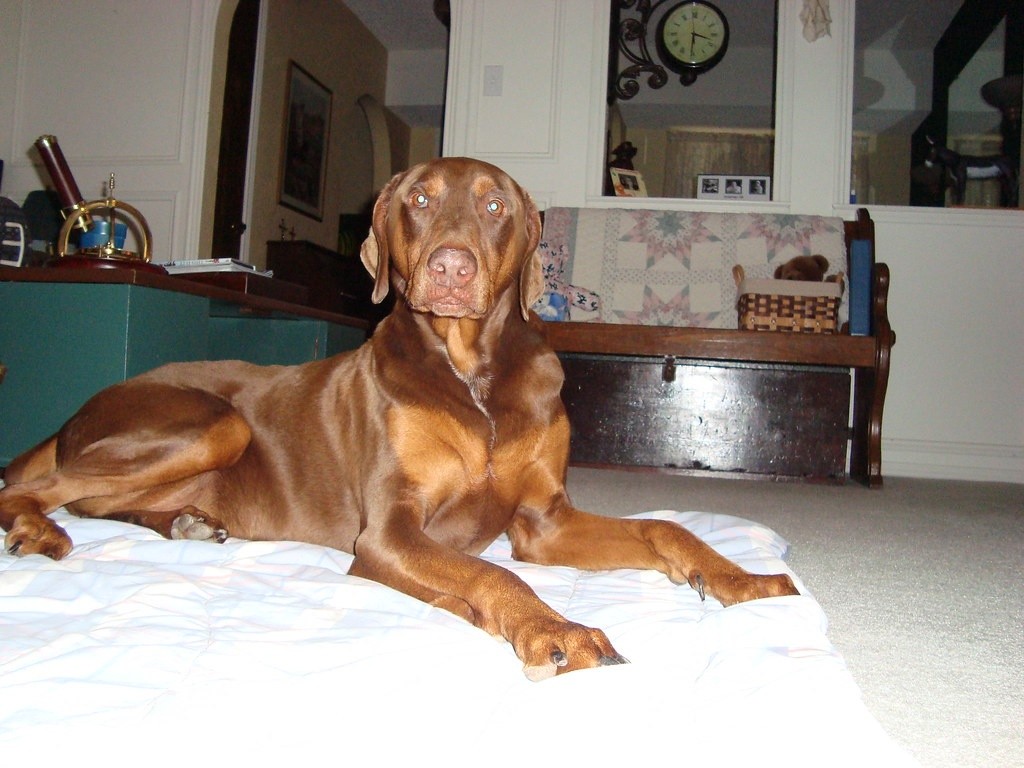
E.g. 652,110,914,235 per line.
0,153,802,681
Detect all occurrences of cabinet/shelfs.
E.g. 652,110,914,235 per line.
0,264,380,478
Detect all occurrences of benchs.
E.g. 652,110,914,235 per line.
528,207,897,490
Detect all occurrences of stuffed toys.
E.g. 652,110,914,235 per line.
774,255,838,283
529,238,601,321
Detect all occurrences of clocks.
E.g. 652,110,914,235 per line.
654,0,730,87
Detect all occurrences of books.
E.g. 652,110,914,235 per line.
149,258,274,278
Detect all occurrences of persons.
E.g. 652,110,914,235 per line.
620,177,639,190
704,180,764,194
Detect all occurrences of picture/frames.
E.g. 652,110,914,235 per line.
275,59,334,224
609,167,649,198
696,173,771,201
